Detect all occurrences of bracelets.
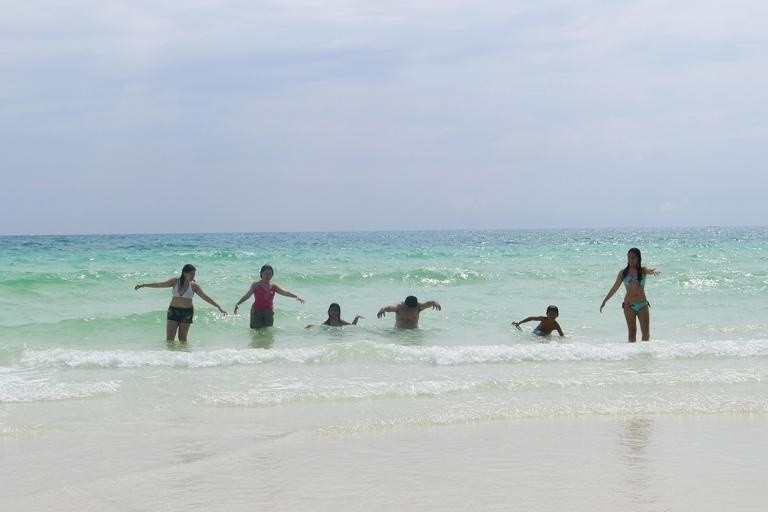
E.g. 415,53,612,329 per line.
143,284,144,287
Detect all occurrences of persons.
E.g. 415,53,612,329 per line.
234,265,306,328
303,303,366,330
600,248,660,342
134,264,228,342
515,305,563,336
377,296,441,330
